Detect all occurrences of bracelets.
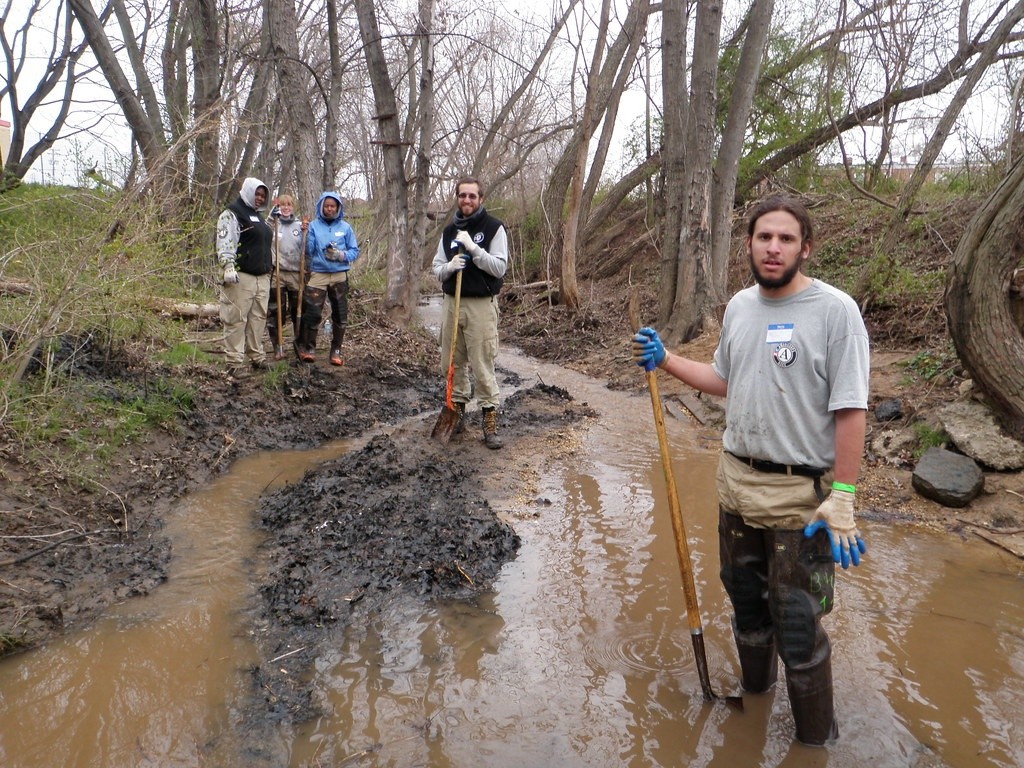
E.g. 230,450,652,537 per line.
832,481,855,492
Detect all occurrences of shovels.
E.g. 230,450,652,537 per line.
272,211,288,360
292,215,307,366
642,334,745,714
429,244,463,445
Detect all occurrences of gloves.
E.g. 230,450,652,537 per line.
223,267,239,284
454,229,478,254
326,248,343,261
632,326,667,372
448,252,470,275
802,483,865,569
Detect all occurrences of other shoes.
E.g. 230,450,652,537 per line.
228,366,253,379
254,359,273,372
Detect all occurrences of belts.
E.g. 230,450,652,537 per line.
725,450,826,478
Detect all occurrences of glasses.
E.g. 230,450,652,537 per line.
455,190,481,200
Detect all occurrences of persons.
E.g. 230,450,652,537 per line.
300,192,359,365
632,200,871,743
432,176,508,447
217,177,282,378
266,195,303,357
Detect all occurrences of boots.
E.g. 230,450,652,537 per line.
482,407,503,450
269,326,288,359
331,324,347,365
452,402,466,434
301,319,319,360
